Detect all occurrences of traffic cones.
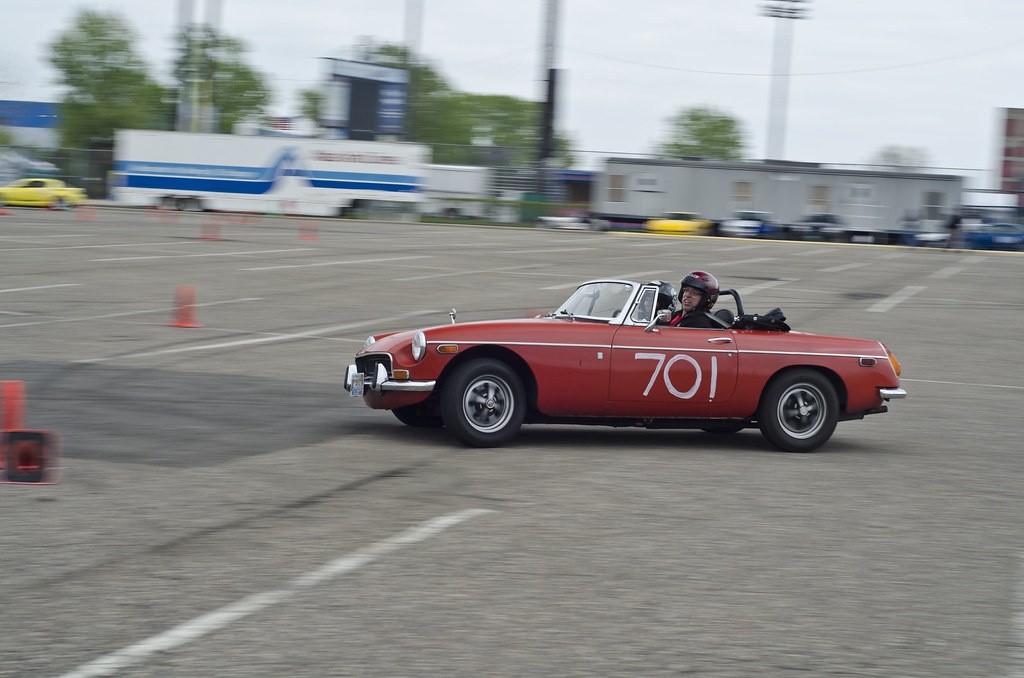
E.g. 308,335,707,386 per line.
1,381,24,471
168,286,205,328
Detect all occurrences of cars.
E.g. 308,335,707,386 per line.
717,212,779,238
789,212,847,240
0,178,87,210
643,212,712,236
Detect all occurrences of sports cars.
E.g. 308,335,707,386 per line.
344,279,908,453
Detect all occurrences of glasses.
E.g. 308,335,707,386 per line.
682,288,702,296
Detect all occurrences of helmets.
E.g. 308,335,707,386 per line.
678,271,719,310
638,280,676,321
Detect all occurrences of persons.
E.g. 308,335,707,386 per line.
637,280,676,325
657,270,720,328
